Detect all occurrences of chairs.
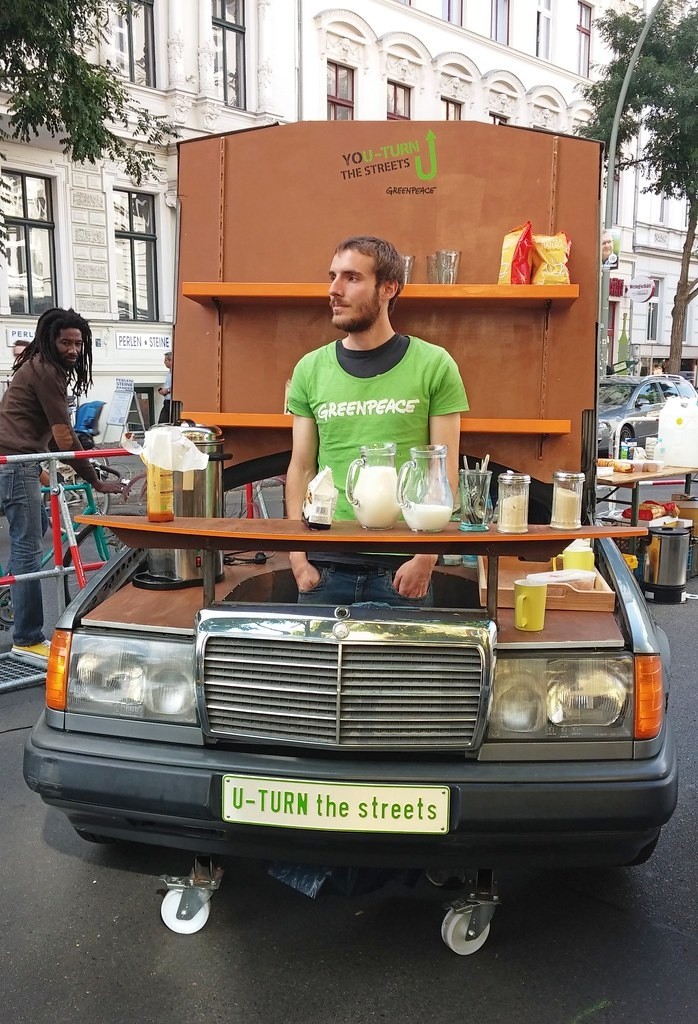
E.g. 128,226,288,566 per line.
73,401,106,436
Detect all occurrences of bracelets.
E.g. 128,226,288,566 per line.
168,389,170,392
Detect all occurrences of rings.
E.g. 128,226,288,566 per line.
121,487,123,490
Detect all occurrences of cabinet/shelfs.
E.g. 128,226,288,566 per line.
172,283,580,461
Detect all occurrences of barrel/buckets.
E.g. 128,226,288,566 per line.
641,526,690,585
133,418,233,592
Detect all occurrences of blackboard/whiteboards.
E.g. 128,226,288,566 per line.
106,391,146,427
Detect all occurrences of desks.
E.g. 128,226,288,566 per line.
73,512,649,633
597,466,698,557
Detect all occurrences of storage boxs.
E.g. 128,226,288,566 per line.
597,516,694,580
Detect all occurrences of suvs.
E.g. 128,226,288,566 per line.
596,374,698,458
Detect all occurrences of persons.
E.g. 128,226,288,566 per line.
158,352,172,423
602,231,619,269
285,236,469,605
13,340,31,363
0,309,132,660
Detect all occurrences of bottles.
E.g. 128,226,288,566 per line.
654,438,666,468
141,452,173,522
498,473,531,534
549,470,586,530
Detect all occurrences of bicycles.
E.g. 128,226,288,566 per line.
37,401,112,540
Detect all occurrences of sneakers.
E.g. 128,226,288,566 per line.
11,639,51,660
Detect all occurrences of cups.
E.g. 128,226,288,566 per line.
458,471,493,533
398,255,415,284
436,250,462,284
345,443,399,531
426,255,437,282
552,547,595,572
396,444,454,533
514,579,547,631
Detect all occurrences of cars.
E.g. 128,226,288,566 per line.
22,116,681,955
